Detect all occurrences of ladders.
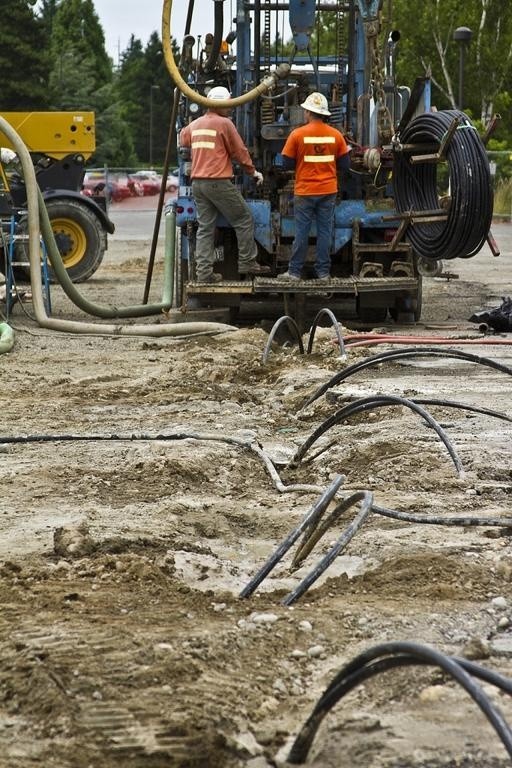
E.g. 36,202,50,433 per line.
6,207,52,319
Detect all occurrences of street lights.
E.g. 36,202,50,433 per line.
453,26,473,108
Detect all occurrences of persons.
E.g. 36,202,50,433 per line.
276,92,351,281
178,86,272,284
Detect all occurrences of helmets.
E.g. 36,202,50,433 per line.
206,85,233,100
299,91,334,117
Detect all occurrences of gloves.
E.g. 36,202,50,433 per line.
252,170,264,186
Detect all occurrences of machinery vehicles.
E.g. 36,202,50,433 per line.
0,111,115,284
163,3,422,323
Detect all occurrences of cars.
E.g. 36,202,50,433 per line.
82,171,179,202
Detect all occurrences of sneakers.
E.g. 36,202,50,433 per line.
197,272,223,284
237,262,272,275
276,269,302,284
